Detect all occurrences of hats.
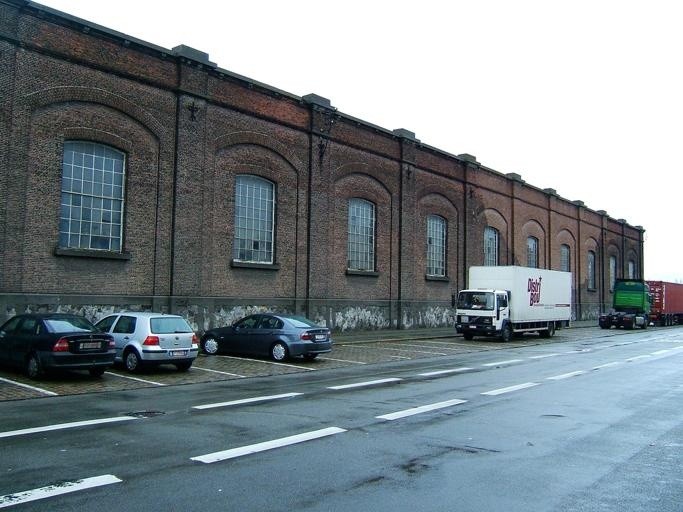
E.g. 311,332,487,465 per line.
200,312,332,362
0,312,199,380
